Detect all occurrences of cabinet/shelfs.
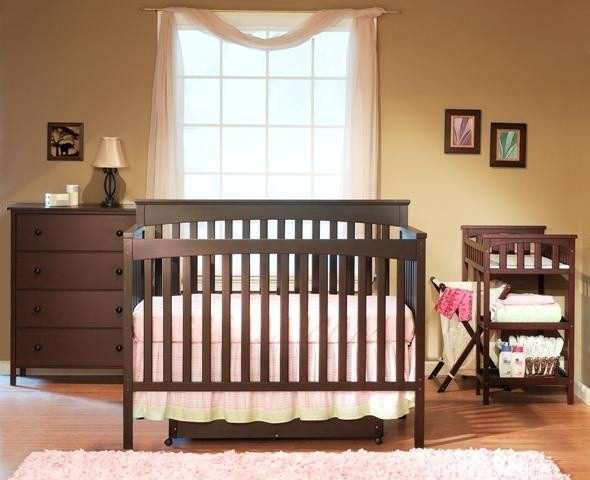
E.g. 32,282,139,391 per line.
460,224,577,406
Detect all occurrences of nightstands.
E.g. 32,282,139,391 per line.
4,202,136,387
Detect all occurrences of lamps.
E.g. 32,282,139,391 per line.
91,136,127,209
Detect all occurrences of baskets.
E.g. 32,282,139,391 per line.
494,335,564,376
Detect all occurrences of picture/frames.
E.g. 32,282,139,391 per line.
47,121,85,161
490,122,527,169
443,108,482,155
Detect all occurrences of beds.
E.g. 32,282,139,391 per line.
120,197,426,449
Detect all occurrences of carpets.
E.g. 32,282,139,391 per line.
8,449,572,480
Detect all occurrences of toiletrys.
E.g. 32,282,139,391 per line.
513,346,527,378
498,342,513,378
